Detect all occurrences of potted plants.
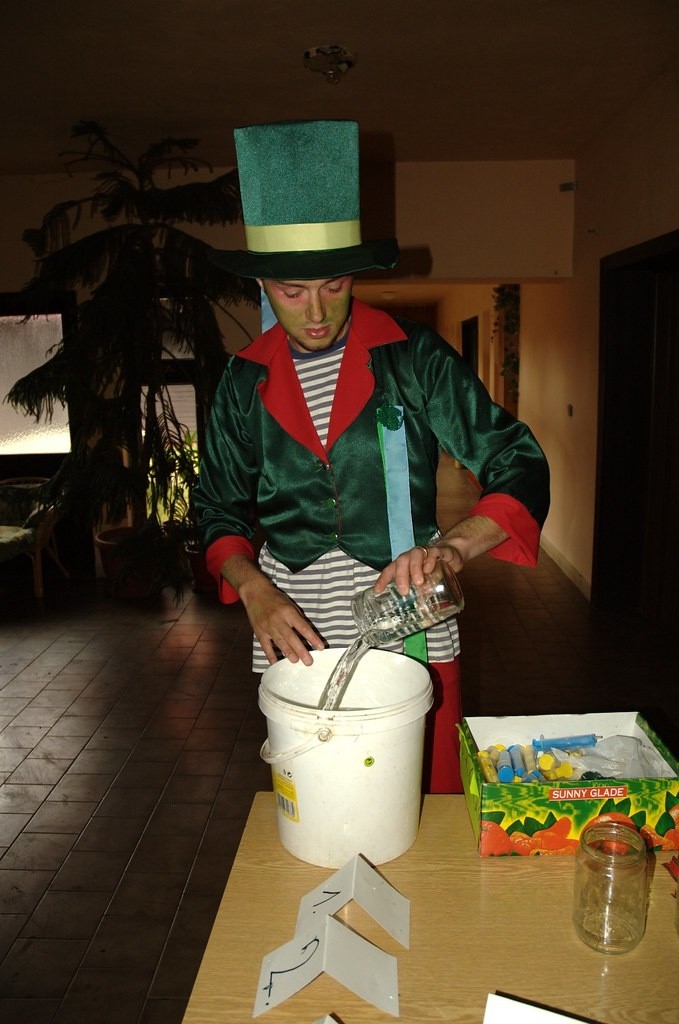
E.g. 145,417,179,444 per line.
10,118,260,604
183,505,218,592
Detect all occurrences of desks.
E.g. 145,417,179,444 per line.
183,791,679,1024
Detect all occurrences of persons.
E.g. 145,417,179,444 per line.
193,210,550,795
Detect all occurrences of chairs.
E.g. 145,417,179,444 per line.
0,477,72,598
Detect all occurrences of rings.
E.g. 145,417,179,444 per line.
412,546,428,561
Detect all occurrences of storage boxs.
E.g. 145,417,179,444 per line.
455,712,679,858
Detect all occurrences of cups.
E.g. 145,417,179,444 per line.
354,562,462,645
574,824,650,954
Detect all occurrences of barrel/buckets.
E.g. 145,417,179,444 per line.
258,648,432,866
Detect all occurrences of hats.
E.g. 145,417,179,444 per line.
206,119,400,281
